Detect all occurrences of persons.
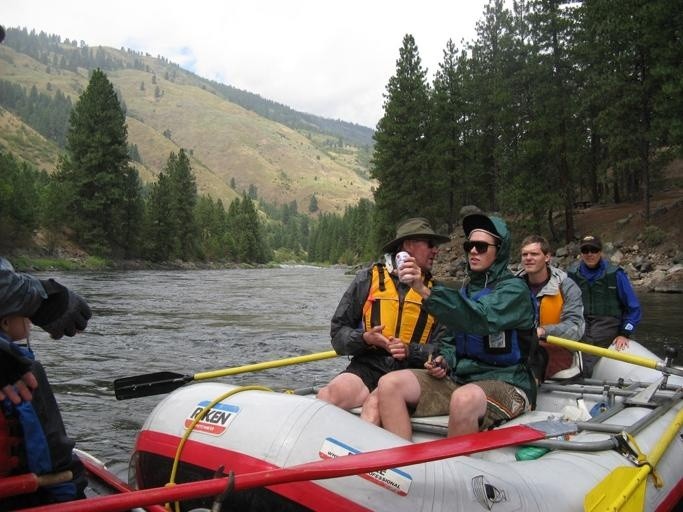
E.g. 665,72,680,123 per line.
315,218,451,426
377,213,537,446
0,314,87,502
512,235,586,383
566,235,641,378
0,269,93,405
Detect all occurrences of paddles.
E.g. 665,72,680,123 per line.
582,409,682,512
113,350,340,401
13,420,577,512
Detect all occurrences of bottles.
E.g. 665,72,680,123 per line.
396,251,414,283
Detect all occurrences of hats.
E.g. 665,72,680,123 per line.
382,217,451,253
580,235,601,250
462,214,500,240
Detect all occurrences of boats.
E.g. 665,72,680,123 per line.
135,338,683,512
0,448,168,512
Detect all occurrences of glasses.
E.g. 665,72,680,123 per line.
581,248,599,254
463,241,498,253
427,240,439,249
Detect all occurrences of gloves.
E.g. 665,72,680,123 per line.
0,337,33,391
29,278,93,340
